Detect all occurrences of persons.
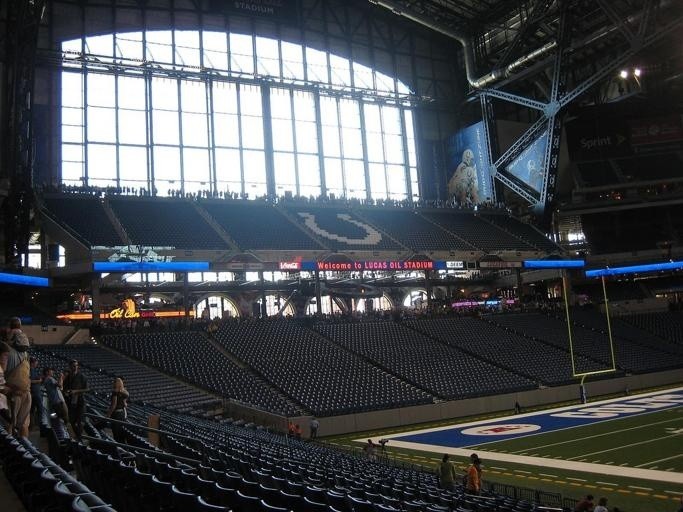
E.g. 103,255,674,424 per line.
93,308,294,333
289,421,304,437
310,418,320,438
463,454,481,496
436,454,456,493
363,439,377,462
512,400,521,415
0,317,90,437
313,295,567,324
574,495,620,512
108,378,130,443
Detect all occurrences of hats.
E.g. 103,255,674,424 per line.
15,332,29,346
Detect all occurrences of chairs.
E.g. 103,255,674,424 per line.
23,308,683,421
45,195,568,255
0,380,567,511
